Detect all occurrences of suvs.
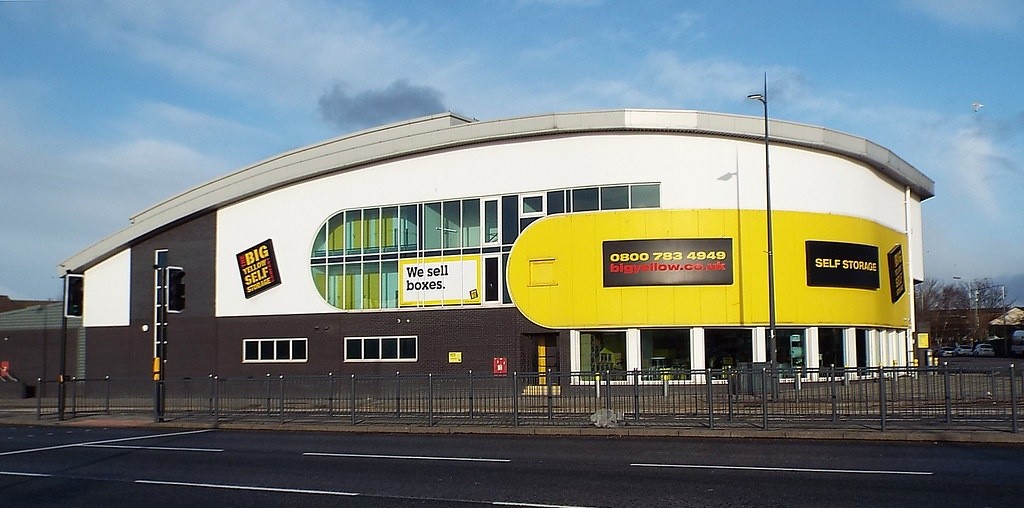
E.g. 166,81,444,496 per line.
1010,330,1024,357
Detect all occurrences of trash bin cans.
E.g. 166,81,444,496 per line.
751,362,779,395
731,361,752,394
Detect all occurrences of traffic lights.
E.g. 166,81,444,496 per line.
64,274,83,318
61,375,70,383
166,266,186,314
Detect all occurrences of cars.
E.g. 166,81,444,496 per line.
937,346,956,357
974,344,995,357
956,345,974,356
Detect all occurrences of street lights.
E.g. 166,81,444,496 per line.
748,71,780,400
953,276,972,327
